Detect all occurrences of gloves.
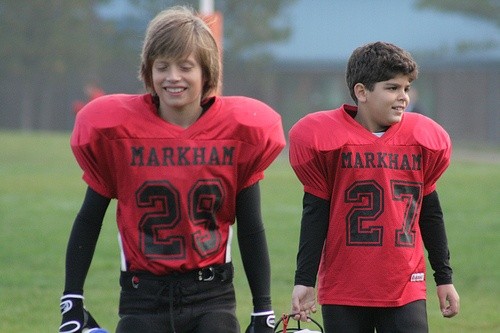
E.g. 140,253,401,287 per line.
57,294,84,333
244,310,276,333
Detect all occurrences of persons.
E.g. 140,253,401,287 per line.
59,5,287,333
288,42,461,333
